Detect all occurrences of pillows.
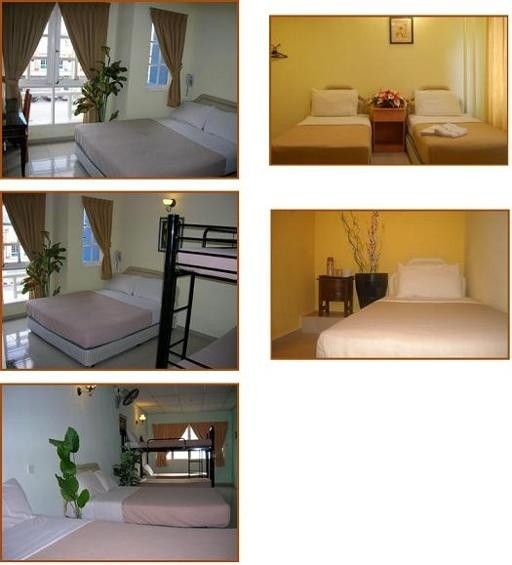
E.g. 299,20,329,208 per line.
77,472,105,496
309,88,358,117
396,262,462,301
207,107,235,144
126,429,137,442
144,463,154,476
3,478,35,530
93,469,118,491
134,280,163,301
413,89,460,116
170,101,213,130
105,272,140,296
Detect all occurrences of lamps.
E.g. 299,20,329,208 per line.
161,198,173,212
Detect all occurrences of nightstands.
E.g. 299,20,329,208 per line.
371,105,407,153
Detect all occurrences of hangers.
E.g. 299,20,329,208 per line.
271,44,288,59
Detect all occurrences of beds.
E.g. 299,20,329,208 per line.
66,462,231,528
156,213,239,369
271,86,372,165
2,478,236,560
119,425,215,488
26,265,176,368
73,94,239,179
315,255,508,358
405,85,477,166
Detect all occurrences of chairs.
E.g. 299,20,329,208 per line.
1,89,33,162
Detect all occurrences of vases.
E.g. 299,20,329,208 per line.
355,272,388,307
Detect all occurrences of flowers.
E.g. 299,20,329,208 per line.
339,210,384,274
369,87,407,107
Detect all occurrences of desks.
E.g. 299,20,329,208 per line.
317,275,354,318
3,110,28,177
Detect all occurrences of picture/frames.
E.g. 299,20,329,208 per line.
157,216,184,254
390,16,414,44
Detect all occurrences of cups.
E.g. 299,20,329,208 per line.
335,267,344,277
